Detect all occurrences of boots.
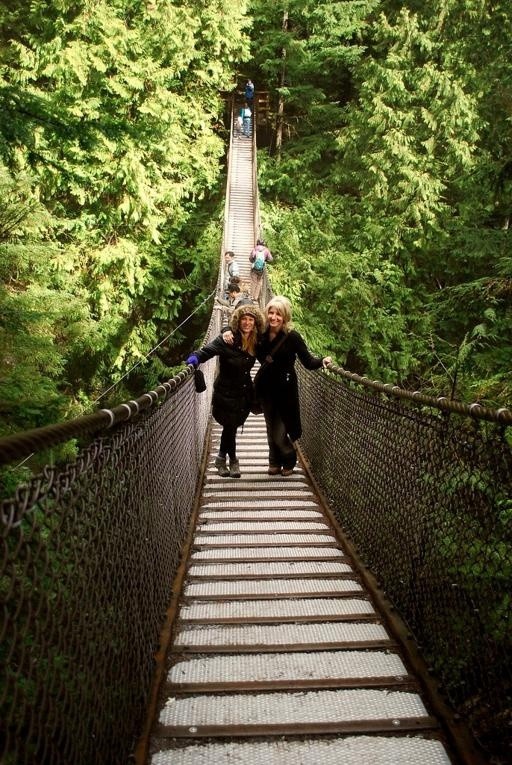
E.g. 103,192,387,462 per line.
216,455,241,478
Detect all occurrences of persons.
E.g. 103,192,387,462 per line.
235,79,255,140
185,238,332,478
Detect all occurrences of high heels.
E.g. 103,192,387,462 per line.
268,465,294,475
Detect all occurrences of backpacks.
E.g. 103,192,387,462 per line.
253,251,264,273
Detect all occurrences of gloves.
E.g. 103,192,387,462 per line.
186,355,198,364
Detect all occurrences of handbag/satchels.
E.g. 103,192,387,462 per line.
194,369,206,393
252,381,263,414
